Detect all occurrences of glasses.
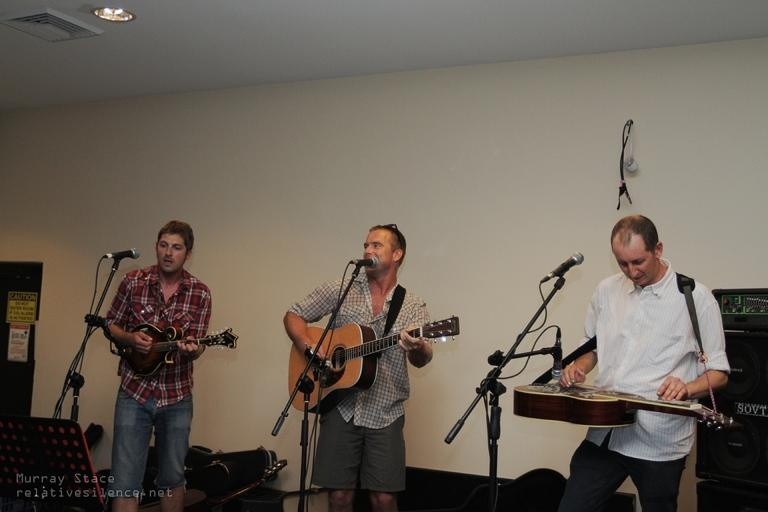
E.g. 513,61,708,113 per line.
370,221,397,233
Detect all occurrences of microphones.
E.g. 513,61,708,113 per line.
540,251,584,284
349,256,379,268
104,248,140,259
551,327,564,381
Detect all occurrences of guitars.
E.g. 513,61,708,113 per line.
513,384,737,429
124,323,239,377
288,317,459,415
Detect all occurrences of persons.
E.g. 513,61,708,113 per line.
558,216,731,512
284,225,433,512
105,221,211,512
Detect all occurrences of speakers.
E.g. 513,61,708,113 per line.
695,331,768,490
697,481,768,512
